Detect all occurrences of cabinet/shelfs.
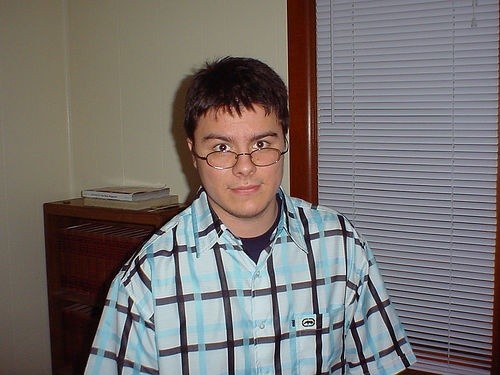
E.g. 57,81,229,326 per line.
43,198,188,375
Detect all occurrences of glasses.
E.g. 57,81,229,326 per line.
193,135,289,170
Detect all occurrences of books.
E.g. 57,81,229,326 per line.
81,186,180,211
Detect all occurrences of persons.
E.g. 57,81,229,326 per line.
83,55,417,375
